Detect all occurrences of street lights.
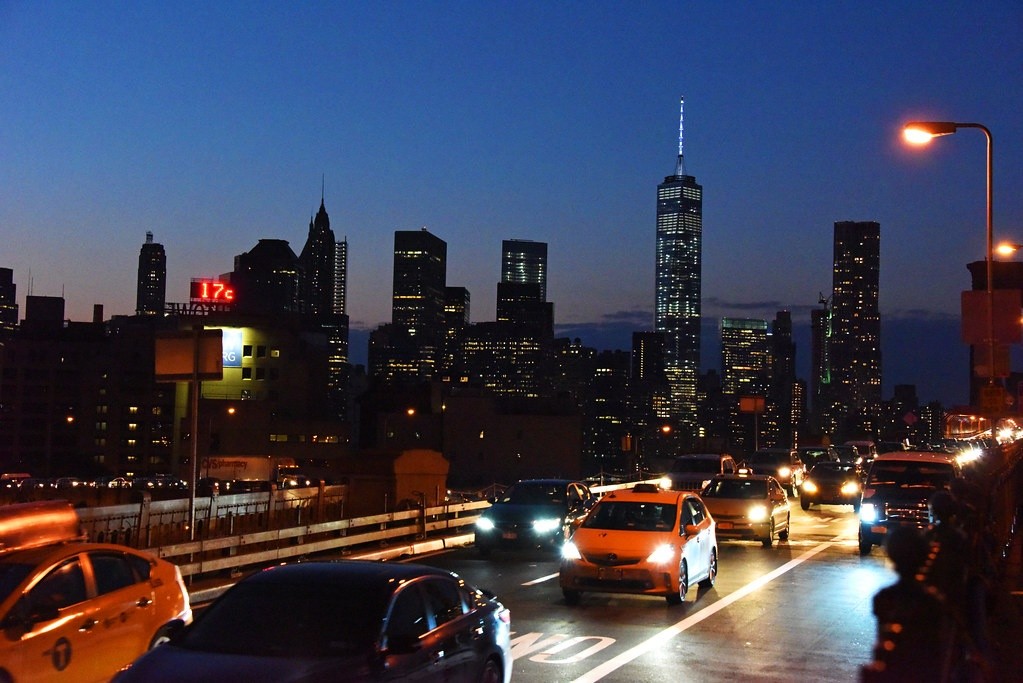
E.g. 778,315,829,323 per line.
381,407,416,511
900,119,997,390
206,406,236,481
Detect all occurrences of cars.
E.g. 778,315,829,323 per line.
557,483,718,605
799,461,864,511
0,458,314,503
107,560,513,683
0,541,193,683
473,478,599,555
700,473,790,549
797,435,999,471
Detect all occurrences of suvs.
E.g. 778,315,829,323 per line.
665,452,738,493
858,451,962,554
746,448,806,495
